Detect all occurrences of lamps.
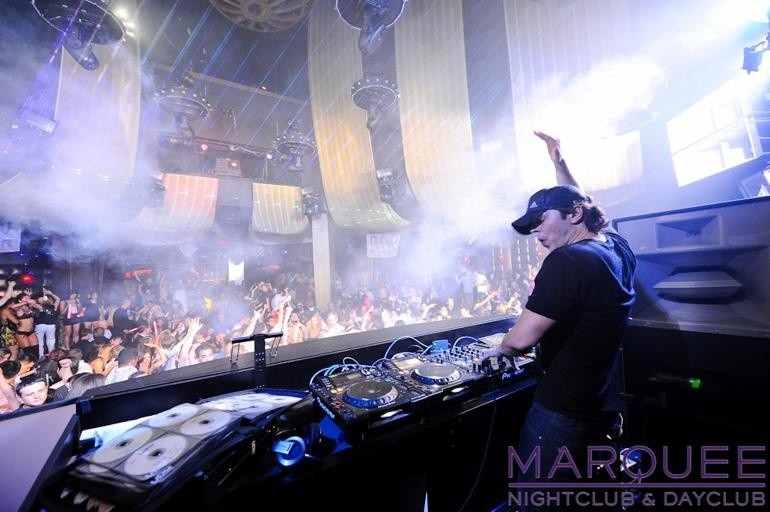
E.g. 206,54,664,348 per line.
2,0,104,176
740,32,770,75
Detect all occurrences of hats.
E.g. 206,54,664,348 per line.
511,185,591,235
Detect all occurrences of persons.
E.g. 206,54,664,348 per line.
1,244,541,422
483,130,639,512
